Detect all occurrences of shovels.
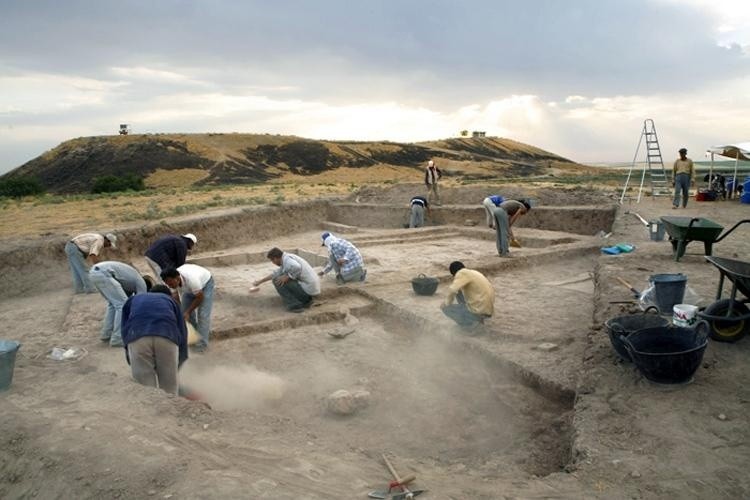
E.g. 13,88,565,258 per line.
368,473,416,499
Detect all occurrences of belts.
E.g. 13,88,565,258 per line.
94,267,99,271
70,241,84,253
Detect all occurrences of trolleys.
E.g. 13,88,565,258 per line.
695,254,750,343
658,214,750,263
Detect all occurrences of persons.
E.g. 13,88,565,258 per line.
439,261,494,336
317,231,367,286
410,197,431,228
495,198,530,257
63,231,214,396
484,195,506,229
425,161,442,204
253,248,320,313
671,149,695,208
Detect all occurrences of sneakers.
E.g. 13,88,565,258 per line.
359,270,366,282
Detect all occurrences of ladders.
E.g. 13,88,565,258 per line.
620,118,672,204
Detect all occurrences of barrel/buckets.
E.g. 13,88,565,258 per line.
649,272,686,315
647,221,664,241
672,304,699,328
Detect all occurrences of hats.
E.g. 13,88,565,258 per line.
181,233,197,244
106,234,117,248
321,232,331,246
142,275,156,292
